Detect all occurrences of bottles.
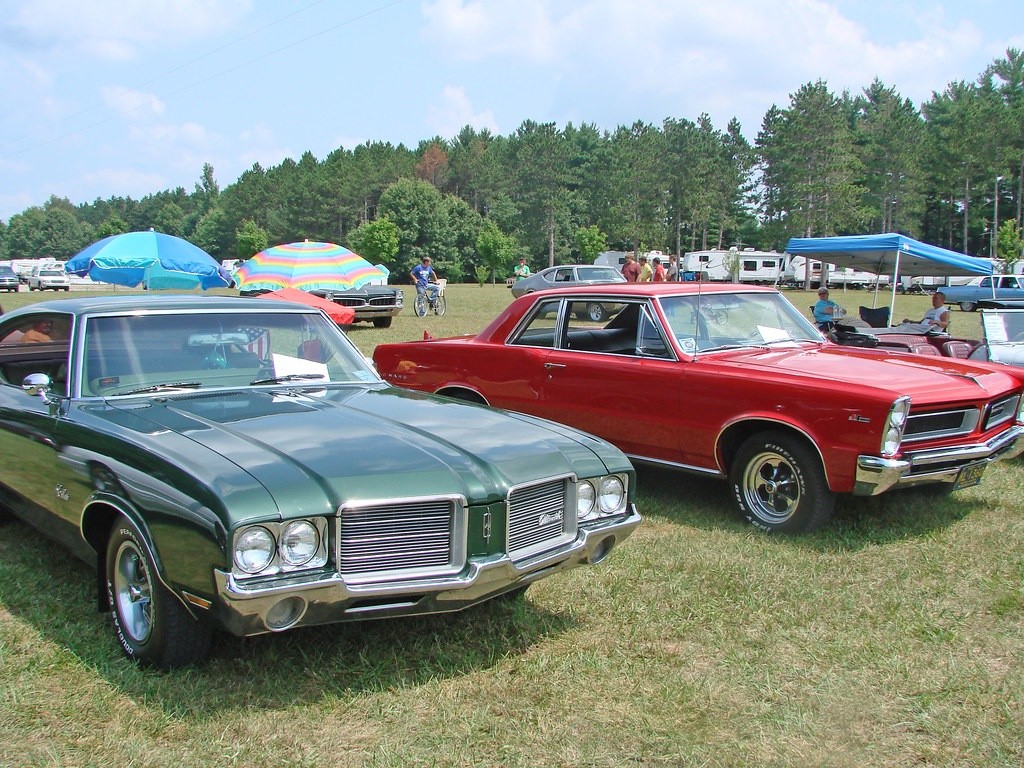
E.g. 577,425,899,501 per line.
838,304,842,318
832,305,838,318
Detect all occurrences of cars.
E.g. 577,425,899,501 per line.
832,307,1023,369
0,295,642,676
27,270,71,291
0,266,21,293
375,284,1024,539
511,265,629,323
240,284,404,328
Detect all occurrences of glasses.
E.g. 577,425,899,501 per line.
818,292,827,295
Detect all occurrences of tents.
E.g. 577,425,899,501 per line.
773,232,996,329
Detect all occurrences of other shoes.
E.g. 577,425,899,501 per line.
430,303,438,308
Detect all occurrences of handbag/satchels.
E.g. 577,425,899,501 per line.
827,323,879,347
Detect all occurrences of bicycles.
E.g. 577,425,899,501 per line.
413,279,447,319
690,304,728,326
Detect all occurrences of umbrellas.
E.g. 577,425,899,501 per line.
64,226,390,291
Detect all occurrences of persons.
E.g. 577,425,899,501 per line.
20,317,55,344
812,287,845,334
514,256,530,281
228,257,245,288
620,254,679,282
903,292,950,333
410,256,440,309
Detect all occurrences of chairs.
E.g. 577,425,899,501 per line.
912,343,941,356
808,306,840,337
520,327,654,353
3,352,260,386
859,305,890,328
943,341,972,358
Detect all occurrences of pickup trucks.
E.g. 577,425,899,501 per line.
936,275,1024,312
